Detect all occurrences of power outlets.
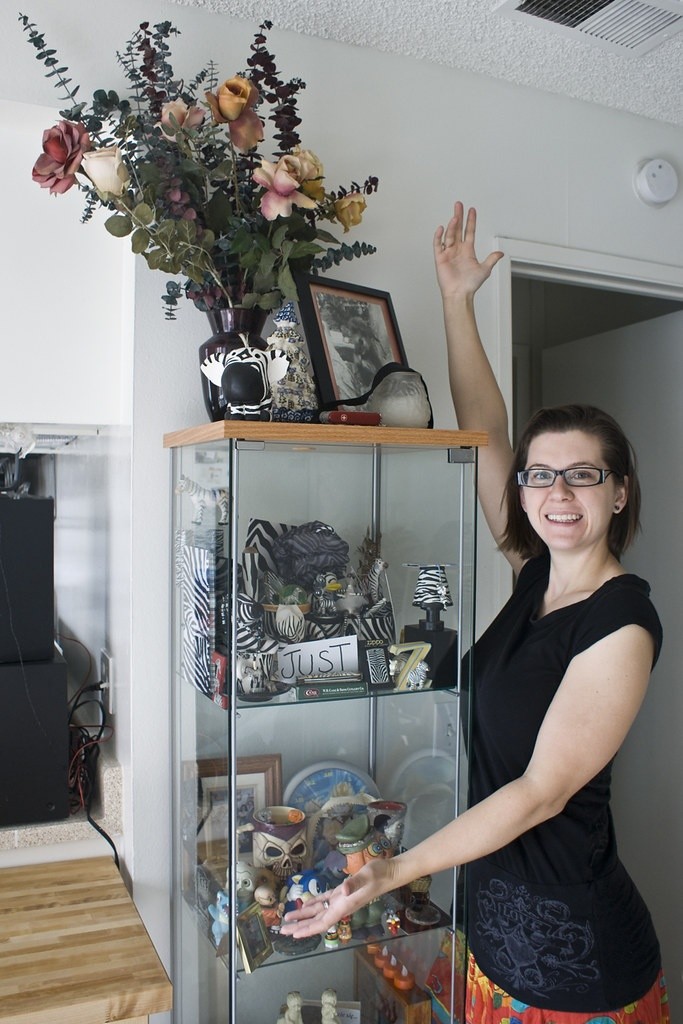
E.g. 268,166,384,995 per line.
99,647,113,716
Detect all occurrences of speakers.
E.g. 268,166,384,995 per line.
0,643,70,830
0,491,55,665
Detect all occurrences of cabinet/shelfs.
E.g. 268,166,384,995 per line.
159,416,491,1023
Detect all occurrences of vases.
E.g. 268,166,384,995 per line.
199,307,274,421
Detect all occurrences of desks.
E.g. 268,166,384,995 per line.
0,856,173,1024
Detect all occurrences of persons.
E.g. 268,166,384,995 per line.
277,202,670,1024
285,991,302,1024
321,988,338,1024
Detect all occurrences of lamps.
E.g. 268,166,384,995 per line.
411,564,454,631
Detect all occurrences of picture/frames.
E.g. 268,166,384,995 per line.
180,752,283,856
236,902,274,974
292,270,410,413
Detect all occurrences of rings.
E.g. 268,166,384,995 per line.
323,900,330,909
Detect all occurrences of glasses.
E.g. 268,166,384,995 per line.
516,467,615,488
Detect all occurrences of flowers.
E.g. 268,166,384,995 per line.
14,8,388,321
